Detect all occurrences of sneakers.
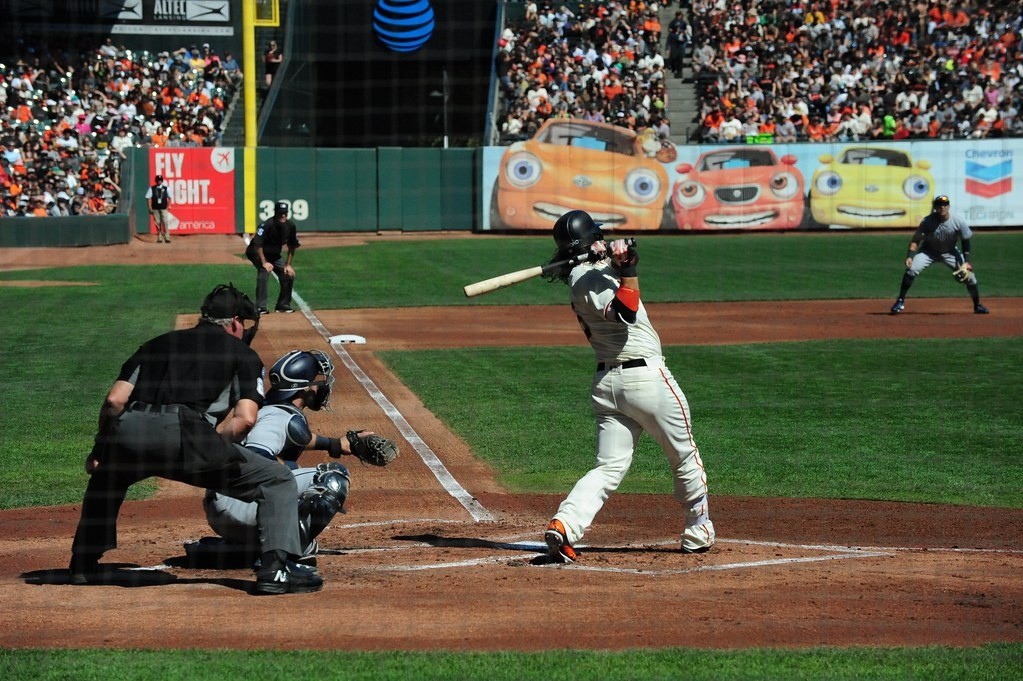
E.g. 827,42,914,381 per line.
543,519,578,564
974,304,990,315
256,558,324,595
891,297,905,314
67,556,102,586
275,306,295,313
256,306,269,314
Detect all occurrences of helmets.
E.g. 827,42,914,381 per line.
274,202,288,213
264,348,336,404
933,195,950,206
551,209,604,256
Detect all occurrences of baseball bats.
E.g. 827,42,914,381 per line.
465,238,638,301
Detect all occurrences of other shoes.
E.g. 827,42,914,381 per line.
164,237,171,242
157,238,163,242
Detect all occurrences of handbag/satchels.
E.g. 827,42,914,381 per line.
179,414,247,477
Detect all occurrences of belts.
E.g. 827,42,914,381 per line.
125,400,184,415
597,358,647,372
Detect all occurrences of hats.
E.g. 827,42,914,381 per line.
0,43,210,206
155,175,163,181
200,281,261,319
527,0,1017,122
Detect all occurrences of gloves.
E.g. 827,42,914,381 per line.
589,239,607,262
608,238,637,278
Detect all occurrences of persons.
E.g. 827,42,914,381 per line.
495,0,672,140
69,283,322,596
183,348,398,570
890,195,989,315
540,211,715,564
0,36,244,216
245,203,299,313
665,0,1023,143
144,175,171,243
265,40,282,87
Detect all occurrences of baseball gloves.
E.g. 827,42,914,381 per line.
347,428,400,469
952,262,970,282
